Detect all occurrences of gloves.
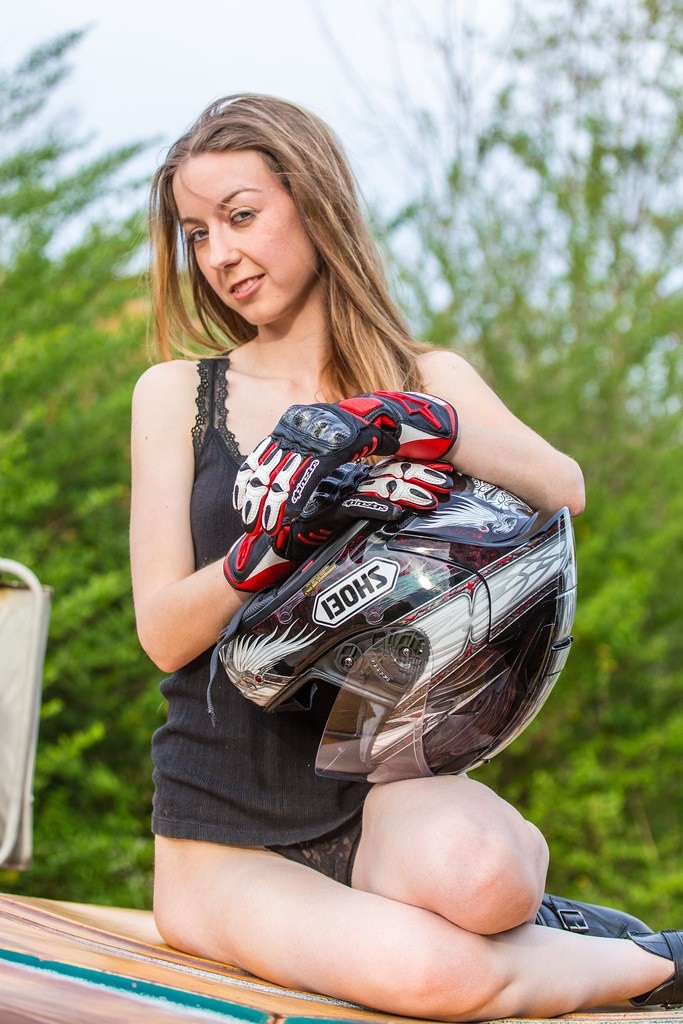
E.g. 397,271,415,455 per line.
223,458,454,603
232,390,459,536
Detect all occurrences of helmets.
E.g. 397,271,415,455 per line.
218,473,577,782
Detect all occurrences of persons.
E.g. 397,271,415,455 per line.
128,92,683,1024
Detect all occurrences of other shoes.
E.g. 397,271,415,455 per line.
627,930,683,1007
535,893,655,939
